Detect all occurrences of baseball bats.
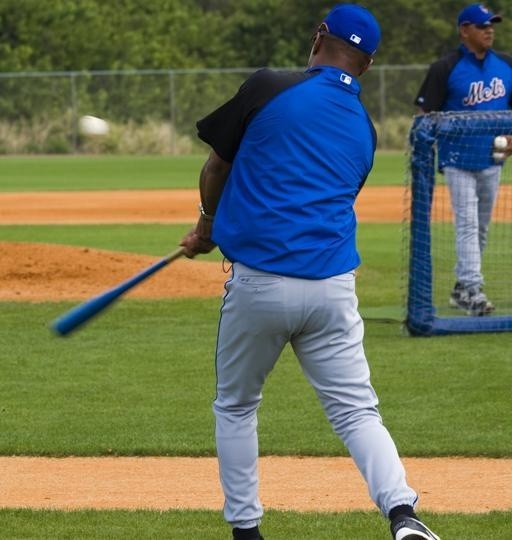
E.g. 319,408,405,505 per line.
49,242,189,337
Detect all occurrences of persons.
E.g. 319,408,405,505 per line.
180,5,439,540
415,3,511,315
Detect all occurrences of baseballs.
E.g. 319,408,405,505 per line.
80,115,110,139
492,136,508,164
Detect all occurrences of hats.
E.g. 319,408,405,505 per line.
457,2,503,27
318,1,382,57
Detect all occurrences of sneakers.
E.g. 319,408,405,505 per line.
449,284,494,317
389,514,441,540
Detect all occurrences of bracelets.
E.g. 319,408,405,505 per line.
199,201,214,221
